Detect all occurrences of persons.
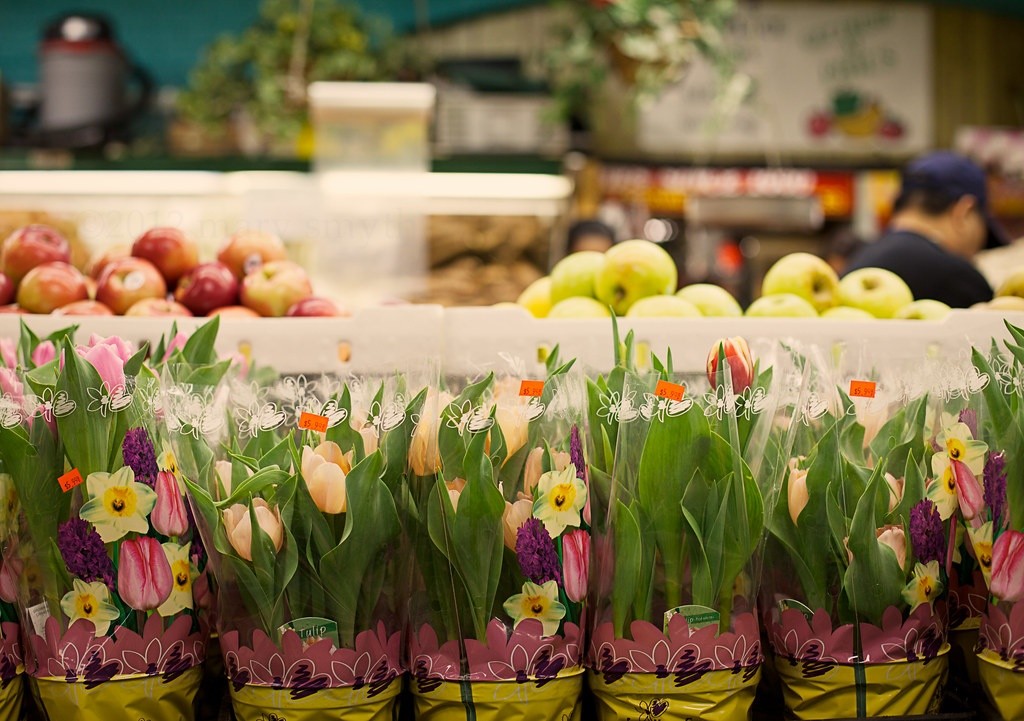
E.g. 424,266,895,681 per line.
841,153,994,310
566,219,617,256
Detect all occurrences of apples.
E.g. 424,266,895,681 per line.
0,225,345,319
744,252,1024,319
491,239,744,317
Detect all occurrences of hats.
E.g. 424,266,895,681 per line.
902,152,1011,247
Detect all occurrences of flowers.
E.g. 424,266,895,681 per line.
0,302,1024,680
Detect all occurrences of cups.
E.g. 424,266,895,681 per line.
36,9,126,143
306,80,433,310
